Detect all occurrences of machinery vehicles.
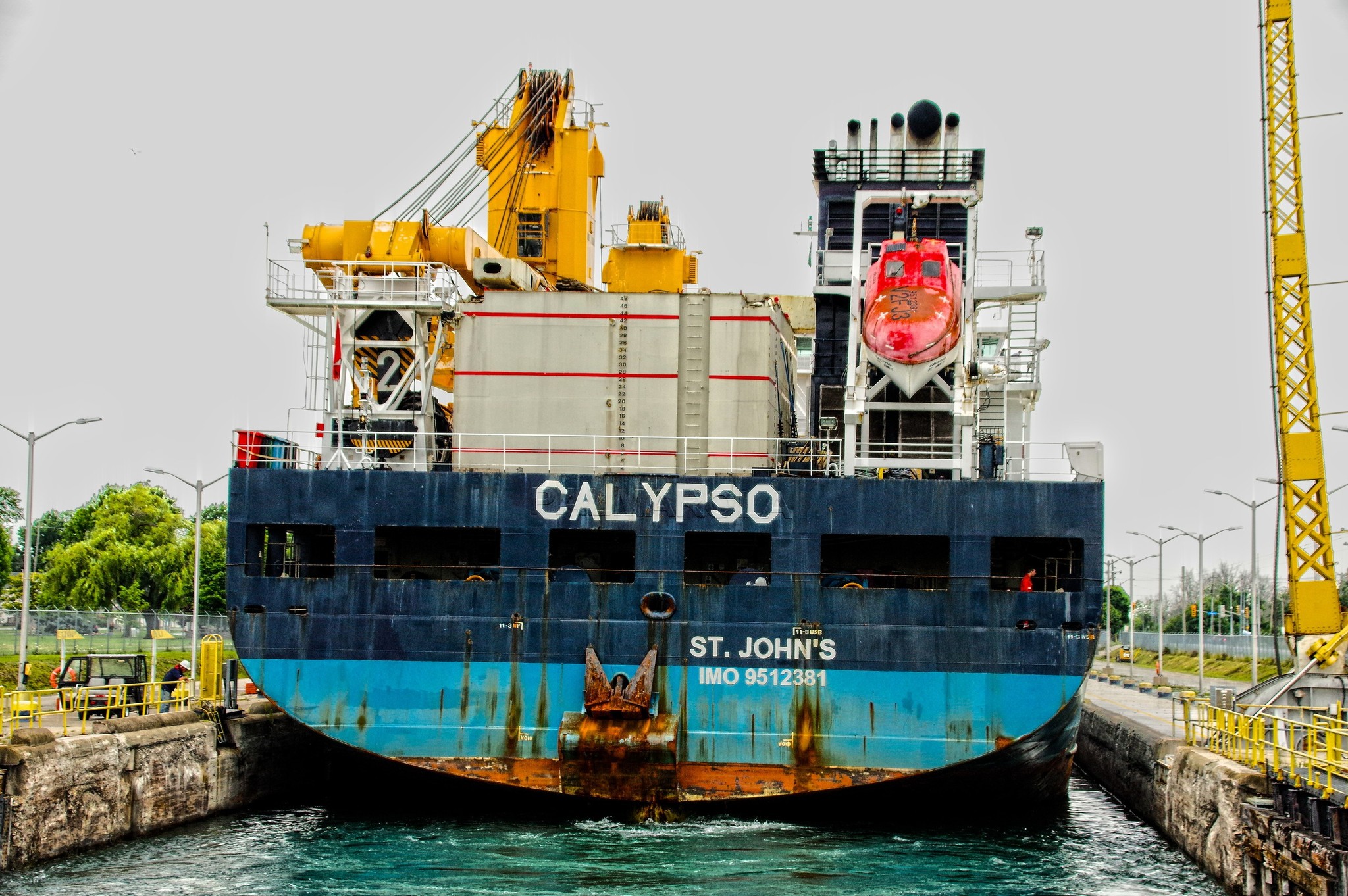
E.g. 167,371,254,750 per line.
1116,645,1135,663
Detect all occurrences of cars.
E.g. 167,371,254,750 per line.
57,653,150,721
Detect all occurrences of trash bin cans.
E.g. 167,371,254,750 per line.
284,439,300,469
272,435,288,468
233,428,266,468
257,434,275,468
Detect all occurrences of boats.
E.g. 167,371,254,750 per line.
865,236,964,399
222,62,1109,815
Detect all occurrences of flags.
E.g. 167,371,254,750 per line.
332,318,342,381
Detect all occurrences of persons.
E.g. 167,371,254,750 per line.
184,624,188,633
1020,567,1037,592
92,625,99,635
109,622,114,632
160,660,191,713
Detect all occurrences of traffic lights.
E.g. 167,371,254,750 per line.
1132,603,1135,611
1191,605,1196,617
1245,606,1249,619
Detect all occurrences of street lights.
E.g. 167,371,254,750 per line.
1203,488,1282,688
1103,555,1136,669
0,418,103,691
1126,530,1197,676
1103,552,1162,678
1235,605,1240,614
1159,523,1244,692
1215,581,1234,635
141,466,230,696
